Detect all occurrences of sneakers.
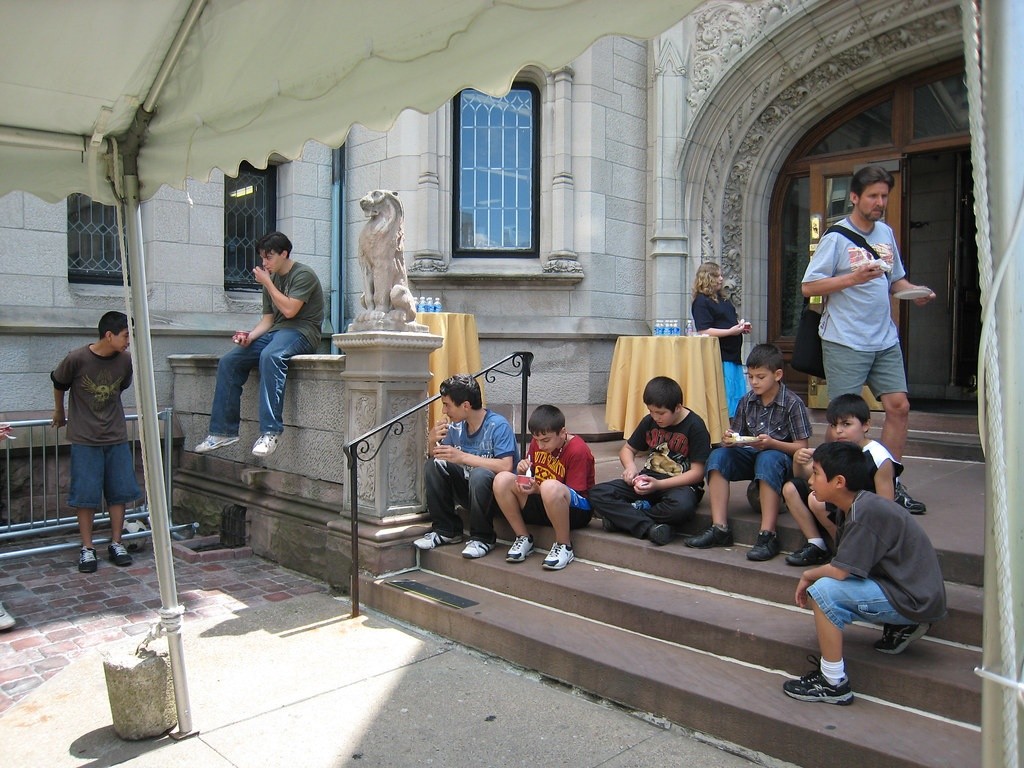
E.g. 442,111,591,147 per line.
252,434,278,456
783,655,853,705
506,534,534,561
462,540,495,558
413,532,462,549
894,482,926,512
108,542,132,565
683,525,733,548
194,435,240,452
786,543,832,566
79,546,97,572
874,622,932,654
747,529,779,560
542,542,574,569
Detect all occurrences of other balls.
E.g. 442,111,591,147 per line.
747,479,787,513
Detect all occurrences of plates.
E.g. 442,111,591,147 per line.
894,288,931,299
728,435,761,443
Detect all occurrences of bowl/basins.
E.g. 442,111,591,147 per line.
235,331,249,342
516,474,534,487
0,424,10,432
744,325,750,330
632,474,649,485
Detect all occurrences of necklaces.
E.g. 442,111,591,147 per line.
278,261,295,295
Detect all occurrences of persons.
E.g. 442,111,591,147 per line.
588,376,711,546
0,425,15,630
692,261,752,424
780,441,948,706
194,231,324,457
493,404,595,570
683,343,808,561
781,392,905,565
800,167,936,513
49,311,133,573
410,374,519,559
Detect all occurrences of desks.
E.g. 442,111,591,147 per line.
419,310,487,452
605,334,733,452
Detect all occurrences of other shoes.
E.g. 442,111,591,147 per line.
0,601,16,629
602,516,617,531
649,523,676,545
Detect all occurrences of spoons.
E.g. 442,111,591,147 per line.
4,434,16,439
526,455,531,477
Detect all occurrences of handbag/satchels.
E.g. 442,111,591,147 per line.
791,309,826,379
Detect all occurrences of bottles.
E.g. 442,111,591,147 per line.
685,320,693,336
434,298,442,312
654,320,680,336
427,297,434,312
419,297,427,312
413,297,419,312
